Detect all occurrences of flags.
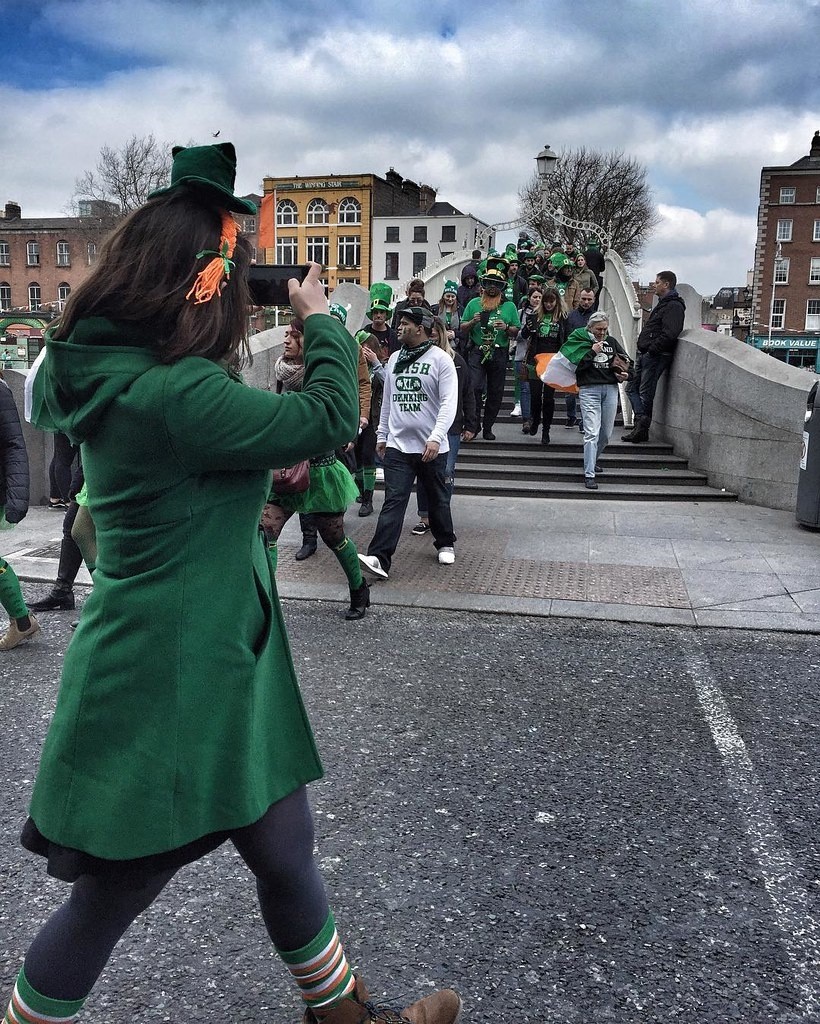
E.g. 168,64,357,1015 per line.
259,192,275,248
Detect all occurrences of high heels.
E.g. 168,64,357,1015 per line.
346,577,370,620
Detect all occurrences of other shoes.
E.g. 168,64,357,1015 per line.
483,429,495,440
522,421,531,434
584,477,598,489
622,429,649,443
530,421,540,435
542,431,550,445
594,465,603,473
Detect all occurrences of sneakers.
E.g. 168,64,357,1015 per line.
0,615,39,651
578,420,585,432
356,553,388,579
565,416,577,429
437,547,455,564
510,404,522,416
411,522,431,535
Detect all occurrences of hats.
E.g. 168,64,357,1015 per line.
479,236,597,288
356,330,370,344
443,280,459,297
329,303,347,325
365,283,392,321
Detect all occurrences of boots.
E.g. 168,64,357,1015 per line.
353,478,373,516
305,971,462,1024
25,539,83,610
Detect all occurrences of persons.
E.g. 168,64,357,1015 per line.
295,303,371,560
2,349,12,369
412,315,477,535
391,231,606,444
354,330,390,516
49,431,78,513
259,317,370,621
355,309,402,480
0,378,40,649
357,307,458,577
540,312,635,489
24,451,85,611
621,271,686,443
70,480,97,630
0,144,460,1024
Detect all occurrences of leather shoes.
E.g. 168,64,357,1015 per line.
295,540,317,559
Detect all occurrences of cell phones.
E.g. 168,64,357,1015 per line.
247,264,308,306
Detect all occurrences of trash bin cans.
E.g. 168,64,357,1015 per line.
794,379,820,531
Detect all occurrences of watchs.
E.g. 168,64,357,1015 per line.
504,324,509,331
